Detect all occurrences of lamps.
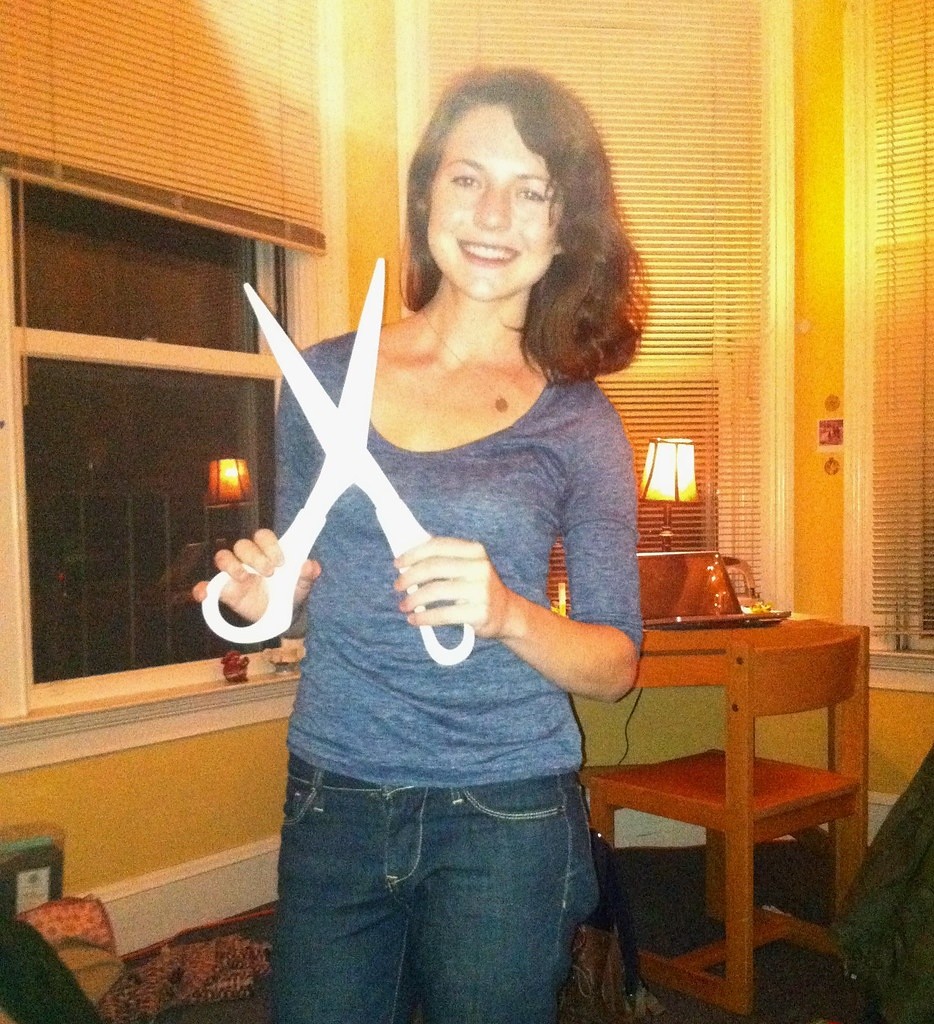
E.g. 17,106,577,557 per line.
205,460,255,534
638,438,700,552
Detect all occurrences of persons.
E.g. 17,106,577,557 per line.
192,69,654,1024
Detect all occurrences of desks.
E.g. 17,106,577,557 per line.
633,621,872,923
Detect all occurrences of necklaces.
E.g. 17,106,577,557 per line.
422,304,529,413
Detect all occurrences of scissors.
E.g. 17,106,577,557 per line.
196,258,479,671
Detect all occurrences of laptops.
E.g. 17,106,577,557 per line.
635,551,792,631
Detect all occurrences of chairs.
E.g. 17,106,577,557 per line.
589,640,857,1016
